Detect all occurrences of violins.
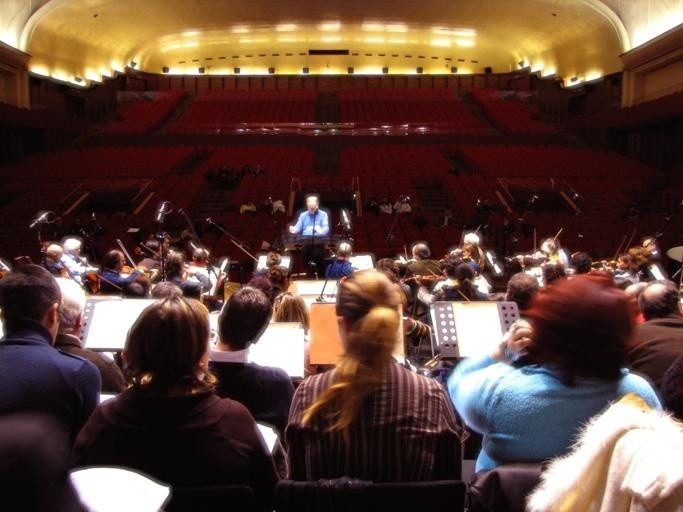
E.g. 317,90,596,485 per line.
593,264,618,273
122,264,153,279
400,271,446,285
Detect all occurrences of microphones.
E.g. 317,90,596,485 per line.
156,204,165,224
205,217,214,225
342,209,352,230
313,207,318,214
28,212,48,229
178,208,186,217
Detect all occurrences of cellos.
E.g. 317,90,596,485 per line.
57,260,71,277
76,254,100,293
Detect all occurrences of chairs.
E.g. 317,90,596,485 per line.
161,484,256,510
480,467,539,510
264,479,468,511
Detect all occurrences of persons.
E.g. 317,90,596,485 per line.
219,164,263,186
288,193,330,278
237,195,258,215
365,195,412,215
1,232,680,511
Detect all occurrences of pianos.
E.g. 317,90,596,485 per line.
283,234,344,249
